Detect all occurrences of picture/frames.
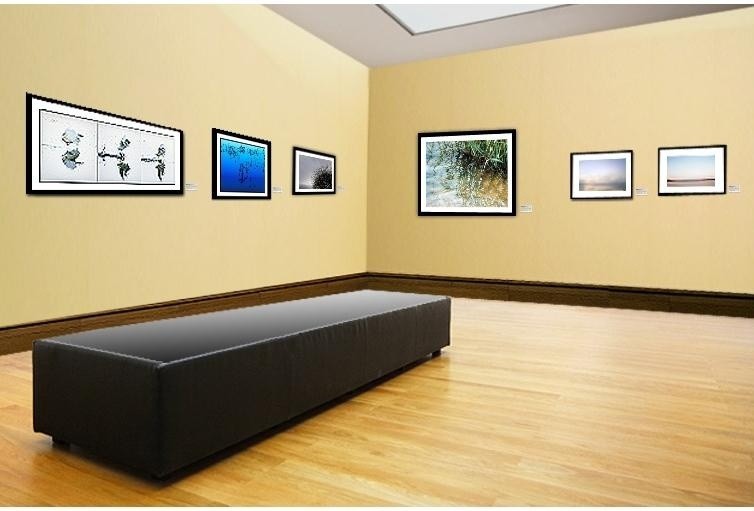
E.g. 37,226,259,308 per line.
570,149,634,200
26,93,186,196
417,129,517,217
212,127,272,199
293,146,337,195
658,144,727,195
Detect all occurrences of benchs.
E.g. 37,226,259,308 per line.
33,288,452,482
